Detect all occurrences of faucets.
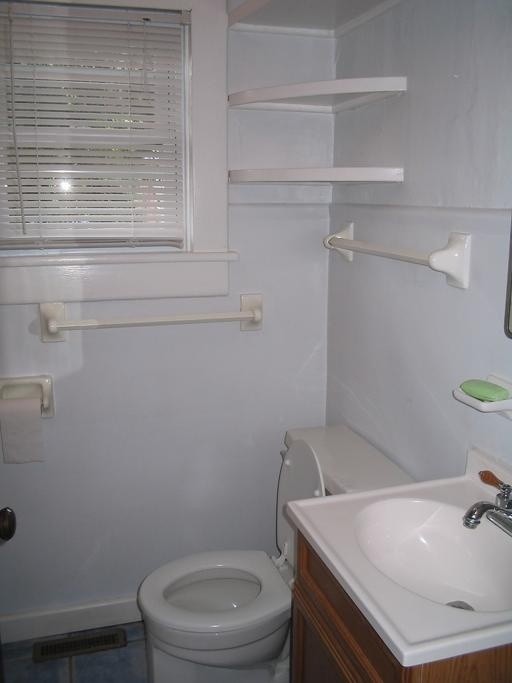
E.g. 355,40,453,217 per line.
461,468,511,541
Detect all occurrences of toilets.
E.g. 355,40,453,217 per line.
137,422,412,682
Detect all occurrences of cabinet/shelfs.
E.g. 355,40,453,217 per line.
225,0,409,187
288,529,512,683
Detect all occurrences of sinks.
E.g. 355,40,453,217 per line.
352,496,512,615
